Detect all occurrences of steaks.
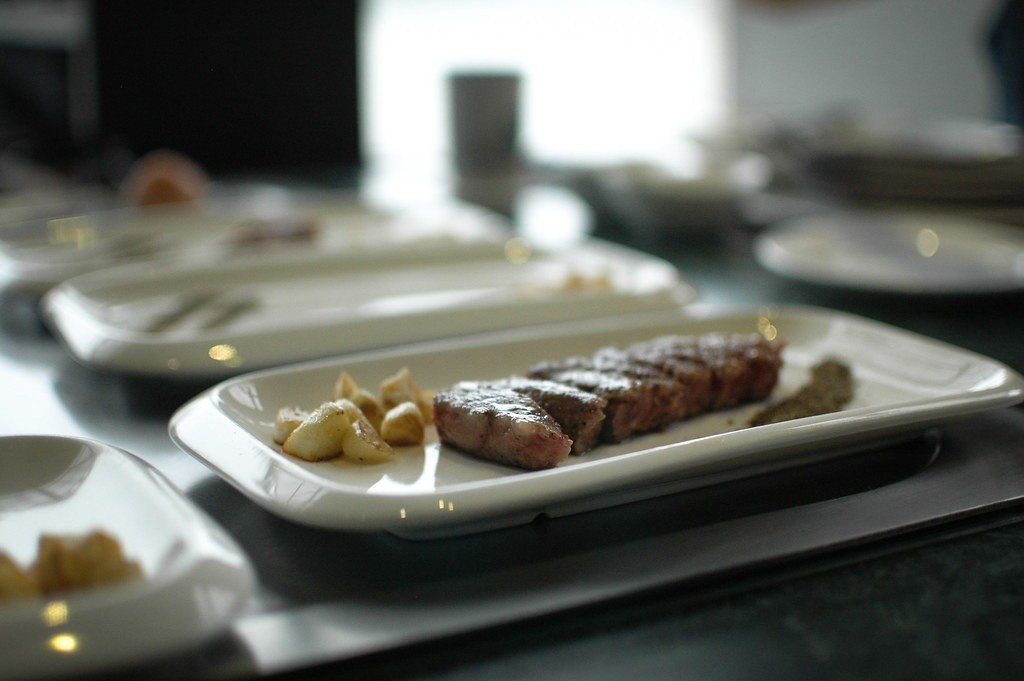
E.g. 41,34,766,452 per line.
433,336,782,467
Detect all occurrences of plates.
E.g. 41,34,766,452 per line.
169,305,1024,535
0,175,693,391
750,210,1024,295
0,431,256,681
